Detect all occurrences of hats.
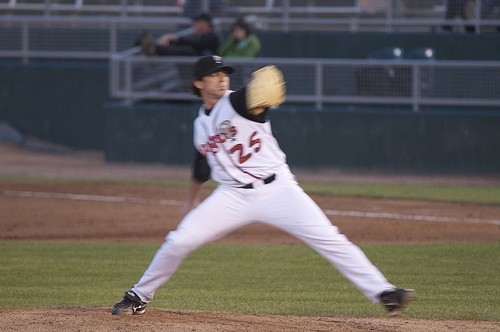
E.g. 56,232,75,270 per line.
192,54,235,76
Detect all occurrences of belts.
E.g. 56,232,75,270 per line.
235,174,276,189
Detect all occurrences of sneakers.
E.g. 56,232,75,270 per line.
111,291,147,315
379,288,417,313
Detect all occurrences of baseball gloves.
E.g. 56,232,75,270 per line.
246,65,286,115
